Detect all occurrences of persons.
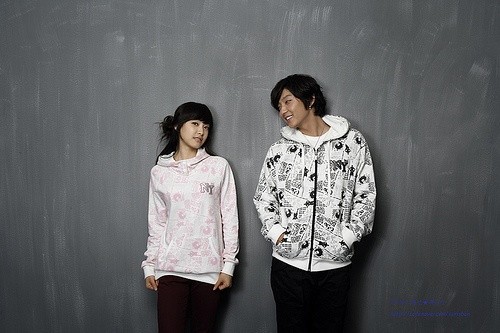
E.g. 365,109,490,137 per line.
140,102,239,333
251,75,377,333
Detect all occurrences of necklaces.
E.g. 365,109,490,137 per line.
300,122,326,151
